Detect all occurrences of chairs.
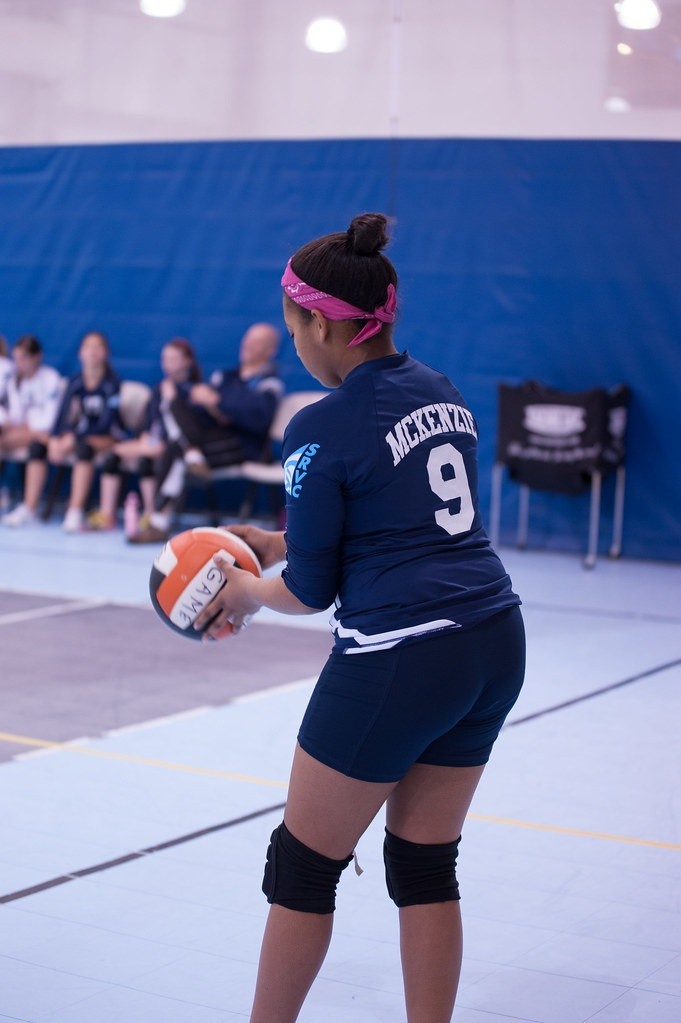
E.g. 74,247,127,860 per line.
40,380,634,571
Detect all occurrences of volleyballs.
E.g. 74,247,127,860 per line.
148,527,265,643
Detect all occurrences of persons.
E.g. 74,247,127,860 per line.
116,335,199,543
0,332,119,532
193,212,528,1023
137,321,286,543
0,336,68,510
83,377,168,529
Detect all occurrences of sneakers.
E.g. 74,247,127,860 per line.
84,513,119,531
129,516,170,541
61,509,89,533
6,504,39,531
